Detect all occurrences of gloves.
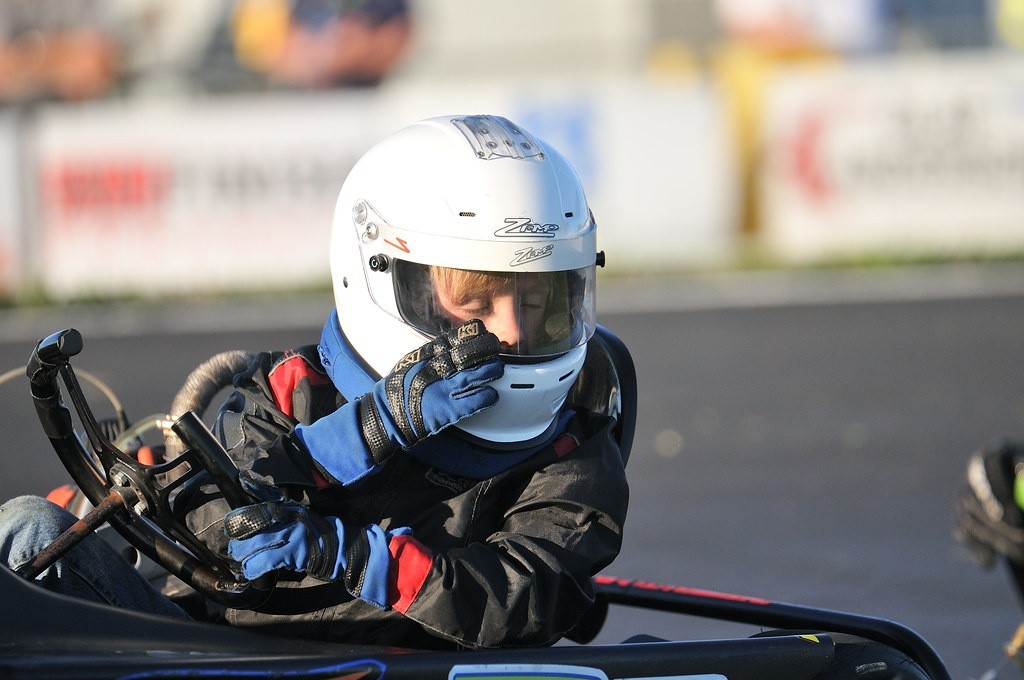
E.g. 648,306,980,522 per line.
295,318,504,487
223,467,413,612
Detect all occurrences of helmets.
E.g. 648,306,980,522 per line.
317,114,606,480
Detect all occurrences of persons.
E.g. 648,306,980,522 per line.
0,115,631,652
